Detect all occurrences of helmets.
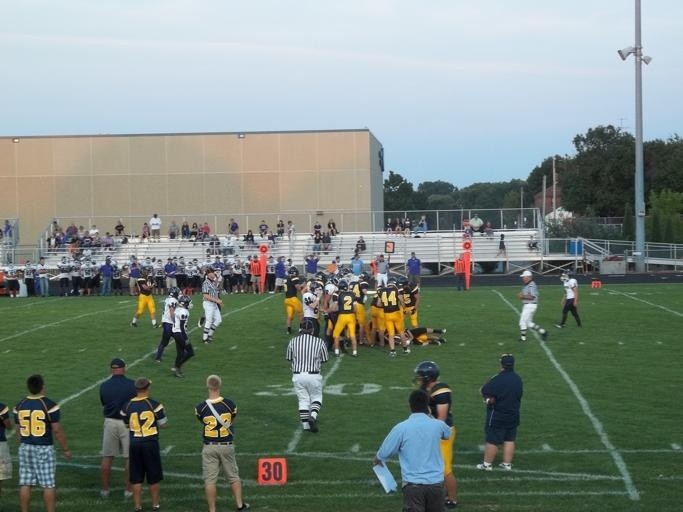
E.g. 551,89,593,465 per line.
559,270,573,282
358,272,370,282
413,360,441,387
310,282,324,296
288,266,300,277
168,287,182,300
178,295,194,310
397,276,409,291
387,278,398,290
337,281,349,292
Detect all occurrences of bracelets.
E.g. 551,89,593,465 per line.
63,446,68,452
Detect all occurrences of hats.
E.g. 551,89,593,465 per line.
519,270,533,277
300,320,314,330
499,353,514,366
110,358,128,369
206,268,217,275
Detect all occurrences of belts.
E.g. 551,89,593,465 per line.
293,371,320,374
204,442,233,446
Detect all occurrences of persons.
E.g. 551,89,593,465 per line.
194,265,226,344
152,287,194,376
552,271,581,329
461,213,496,240
494,234,508,258
455,253,468,290
528,236,540,251
0,214,423,295
415,361,458,508
0,403,12,481
518,271,547,342
286,319,329,433
129,265,163,330
372,391,452,512
476,353,523,471
100,358,137,499
13,375,70,512
383,215,431,238
277,263,450,357
194,375,250,512
119,377,168,512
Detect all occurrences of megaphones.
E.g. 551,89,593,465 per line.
618,46,634,60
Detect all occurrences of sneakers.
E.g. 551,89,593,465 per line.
170,366,185,380
498,462,512,472
438,327,446,334
349,352,359,358
151,504,162,512
198,318,204,328
307,416,319,433
130,322,139,328
437,335,448,344
201,338,212,345
123,490,134,499
552,322,564,329
133,505,144,512
402,346,411,354
332,351,340,358
542,331,548,341
388,350,398,359
444,499,457,509
476,462,493,472
152,324,161,329
517,338,528,343
299,421,310,432
236,502,250,512
208,336,215,342
100,488,110,498
